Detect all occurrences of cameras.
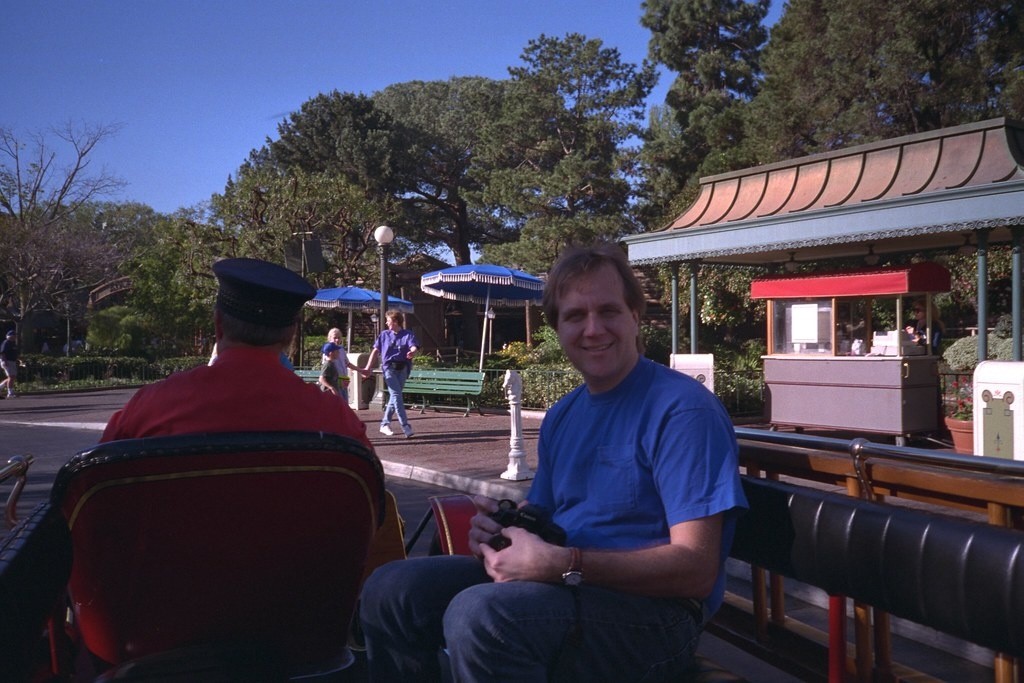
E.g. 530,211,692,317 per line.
485,502,566,552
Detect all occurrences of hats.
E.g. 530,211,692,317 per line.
324,342,343,357
211,256,320,327
7,331,19,336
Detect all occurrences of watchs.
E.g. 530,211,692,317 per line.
562,547,586,590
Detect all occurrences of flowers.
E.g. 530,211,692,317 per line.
945,376,972,422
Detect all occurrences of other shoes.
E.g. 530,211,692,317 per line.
7,393,18,399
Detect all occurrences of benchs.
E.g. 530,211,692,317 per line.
380,370,486,416
684,425,1024,683
295,369,321,384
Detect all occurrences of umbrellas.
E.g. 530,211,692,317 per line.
304,285,415,352
421,264,545,373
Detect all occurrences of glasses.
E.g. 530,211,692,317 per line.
912,308,926,314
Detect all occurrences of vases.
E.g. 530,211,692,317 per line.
945,415,973,456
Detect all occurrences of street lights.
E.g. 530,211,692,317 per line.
63,297,72,358
487,308,495,355
373,225,395,333
371,310,378,343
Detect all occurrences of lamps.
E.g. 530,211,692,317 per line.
488,307,496,319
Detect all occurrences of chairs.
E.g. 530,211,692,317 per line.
35,432,388,683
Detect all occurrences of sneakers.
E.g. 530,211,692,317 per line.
380,425,395,436
402,425,415,439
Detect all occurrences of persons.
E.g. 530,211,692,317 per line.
96,260,376,454
318,309,420,439
358,248,750,683
887,298,946,447
0,330,23,399
